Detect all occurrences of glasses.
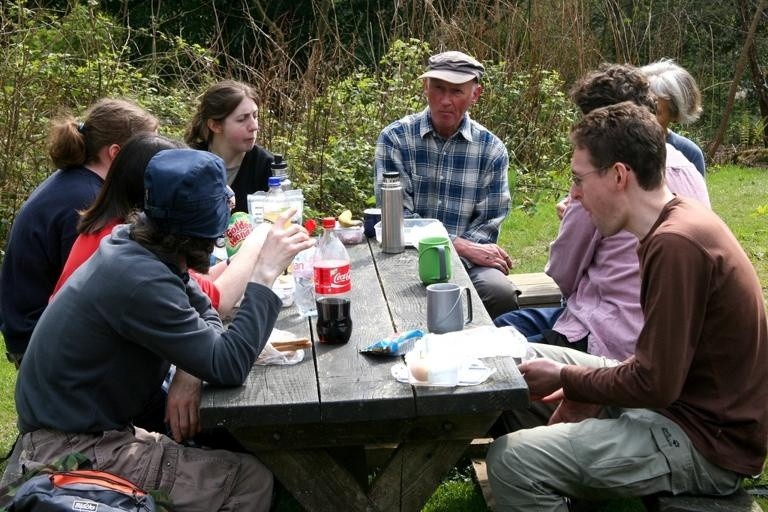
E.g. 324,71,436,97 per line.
572,161,630,186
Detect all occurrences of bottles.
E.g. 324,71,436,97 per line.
313,217,352,344
381,171,404,254
264,176,287,197
268,155,292,192
292,217,321,318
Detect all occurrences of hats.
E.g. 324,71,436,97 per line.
138,149,233,239
416,51,483,86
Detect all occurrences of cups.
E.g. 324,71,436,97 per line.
426,282,473,334
364,208,381,237
418,237,451,286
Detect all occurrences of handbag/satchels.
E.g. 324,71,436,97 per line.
12,470,156,512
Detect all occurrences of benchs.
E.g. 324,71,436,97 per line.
646,488,764,512
506,272,563,309
1,431,24,512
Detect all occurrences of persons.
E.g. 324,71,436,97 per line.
372,49,521,320
636,55,706,178
486,99,768,512
15,147,318,509
0,97,160,371
185,80,275,215
48,131,275,323
493,61,711,361
